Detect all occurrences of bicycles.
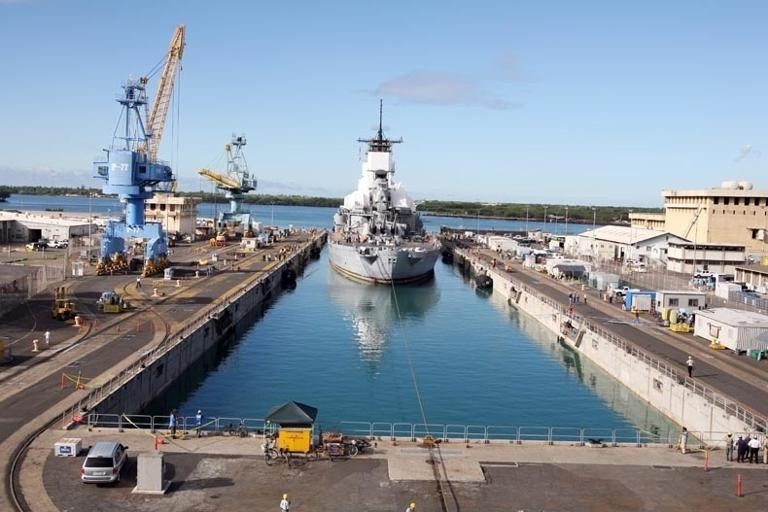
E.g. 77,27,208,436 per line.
262,434,359,466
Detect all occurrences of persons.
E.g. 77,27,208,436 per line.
724,431,767,464
678,425,688,455
634,309,640,323
686,354,694,376
405,501,417,512
135,275,142,289
195,409,204,438
168,408,179,439
43,329,51,344
279,493,291,512
568,290,579,305
583,293,587,304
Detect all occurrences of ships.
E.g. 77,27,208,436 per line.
327,99,443,287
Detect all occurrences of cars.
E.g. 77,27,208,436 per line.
81,442,128,483
